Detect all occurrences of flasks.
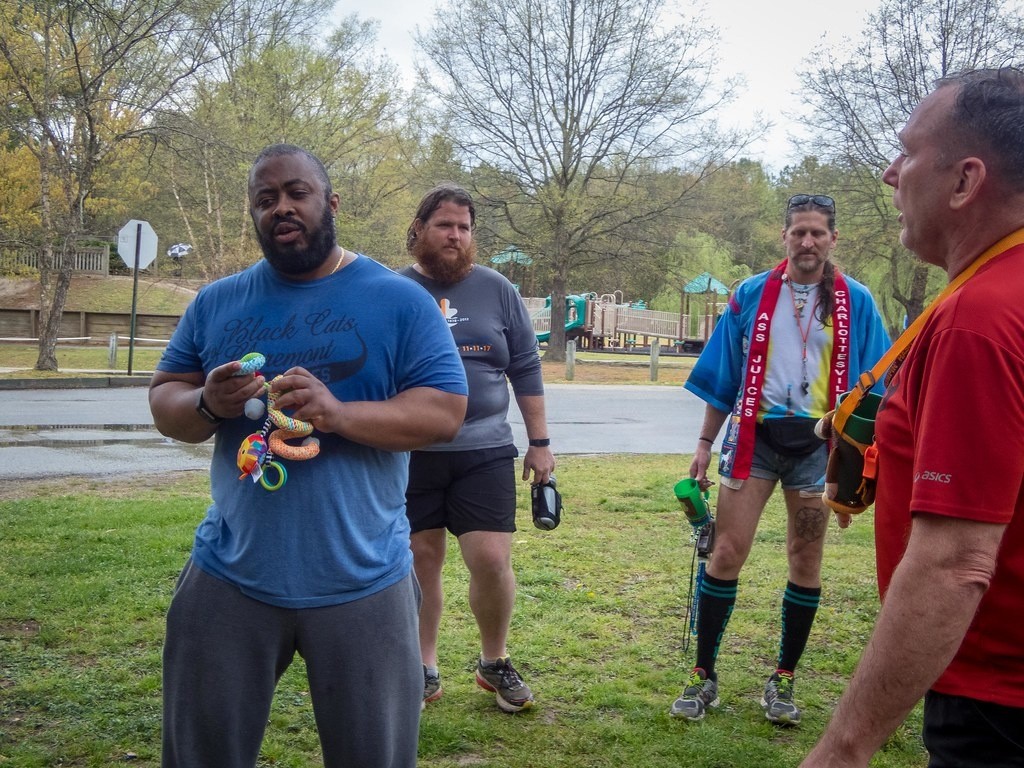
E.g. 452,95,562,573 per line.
531,477,562,530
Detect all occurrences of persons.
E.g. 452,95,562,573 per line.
798,68,1024,768
397,188,556,711
669,195,892,726
172,256,183,279
149,145,468,768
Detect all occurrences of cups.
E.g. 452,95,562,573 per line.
822,392,883,514
674,478,710,526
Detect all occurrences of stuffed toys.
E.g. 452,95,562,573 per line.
232,353,319,491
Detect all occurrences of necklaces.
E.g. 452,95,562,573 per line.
331,247,344,273
784,281,819,395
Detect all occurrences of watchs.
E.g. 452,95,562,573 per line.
195,389,224,425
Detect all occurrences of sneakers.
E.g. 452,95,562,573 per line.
422,663,442,709
476,656,533,712
669,667,720,720
761,670,801,725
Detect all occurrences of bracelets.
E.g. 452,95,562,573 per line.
529,439,550,447
699,437,714,444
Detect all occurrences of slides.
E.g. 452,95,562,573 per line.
535,322,578,343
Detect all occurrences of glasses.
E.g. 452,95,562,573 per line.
787,194,835,212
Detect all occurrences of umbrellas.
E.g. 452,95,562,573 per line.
167,242,193,258
